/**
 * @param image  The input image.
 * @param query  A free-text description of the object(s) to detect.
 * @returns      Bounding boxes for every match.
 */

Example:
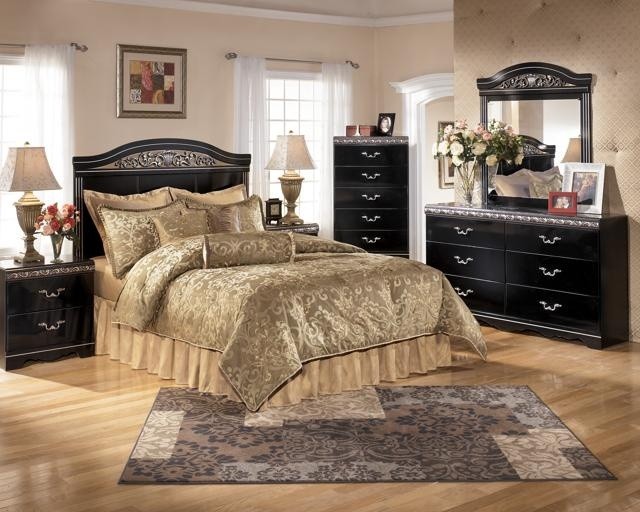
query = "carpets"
[116,384,618,488]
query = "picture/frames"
[376,112,395,136]
[437,121,455,190]
[114,42,189,119]
[546,162,607,219]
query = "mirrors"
[476,59,595,203]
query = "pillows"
[82,182,267,278]
[490,166,563,200]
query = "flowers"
[488,119,524,165]
[31,200,82,248]
[433,120,497,171]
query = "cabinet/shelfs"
[333,134,412,259]
[423,203,630,350]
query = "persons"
[576,177,591,204]
[561,197,572,209]
[554,199,563,209]
[382,117,390,133]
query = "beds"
[65,136,446,410]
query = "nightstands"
[0,259,95,369]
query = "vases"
[49,235,66,264]
[453,163,475,205]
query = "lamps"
[0,141,63,269]
[265,132,317,224]
